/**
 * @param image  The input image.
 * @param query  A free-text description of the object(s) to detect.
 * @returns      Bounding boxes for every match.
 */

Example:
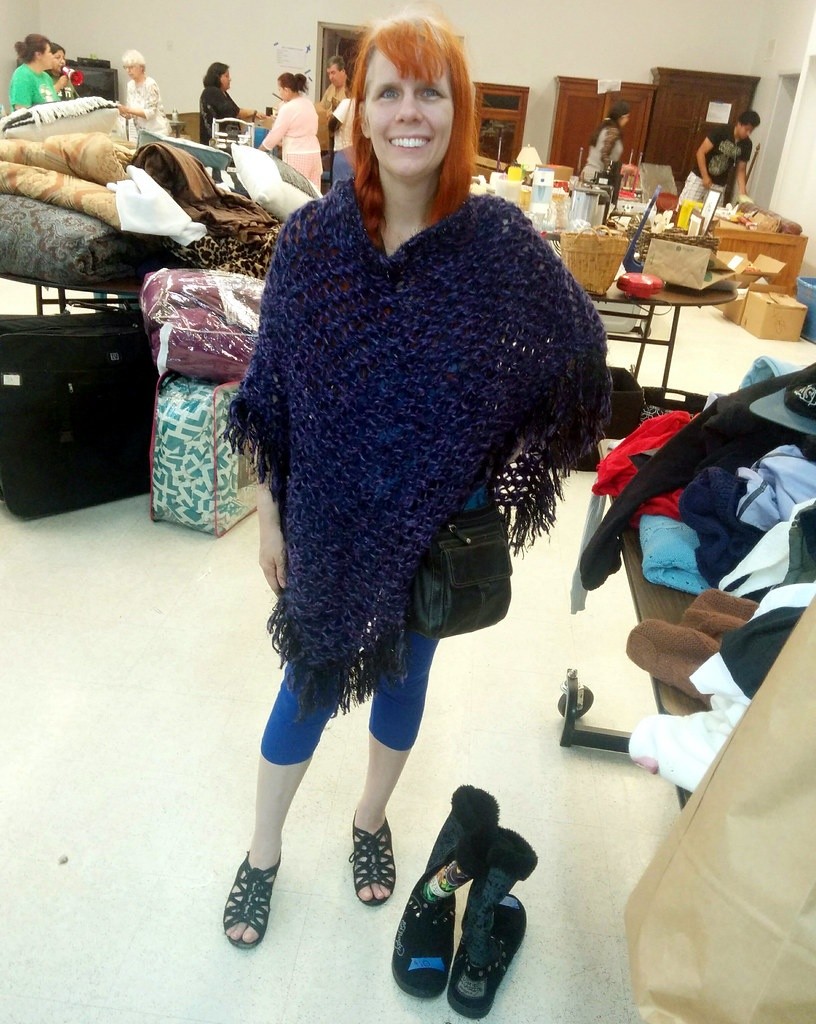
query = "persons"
[315,54,356,188]
[199,62,267,148]
[117,50,172,140]
[220,11,613,950]
[45,42,80,101]
[257,72,323,193]
[676,110,760,214]
[581,100,631,209]
[8,33,60,114]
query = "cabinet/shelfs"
[643,67,762,209]
[473,81,529,165]
[548,77,656,175]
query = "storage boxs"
[551,367,645,473]
[716,249,816,344]
[535,164,574,182]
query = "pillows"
[0,96,121,142]
[231,142,324,221]
[136,130,231,170]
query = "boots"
[448,826,538,1019]
[392,785,499,999]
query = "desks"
[589,273,738,388]
[1,274,142,316]
[558,440,712,811]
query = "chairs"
[643,386,707,414]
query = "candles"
[498,137,501,160]
[636,152,643,173]
[628,149,634,165]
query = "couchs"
[707,202,809,297]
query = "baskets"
[561,225,629,296]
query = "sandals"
[348,811,395,907]
[223,847,282,949]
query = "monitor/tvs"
[73,66,119,103]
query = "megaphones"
[61,66,84,86]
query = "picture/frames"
[700,188,723,236]
[688,210,705,237]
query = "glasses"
[123,65,138,70]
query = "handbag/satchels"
[142,265,267,380]
[408,504,512,637]
[151,370,258,537]
[1,298,158,522]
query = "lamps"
[517,144,542,172]
[577,147,583,173]
